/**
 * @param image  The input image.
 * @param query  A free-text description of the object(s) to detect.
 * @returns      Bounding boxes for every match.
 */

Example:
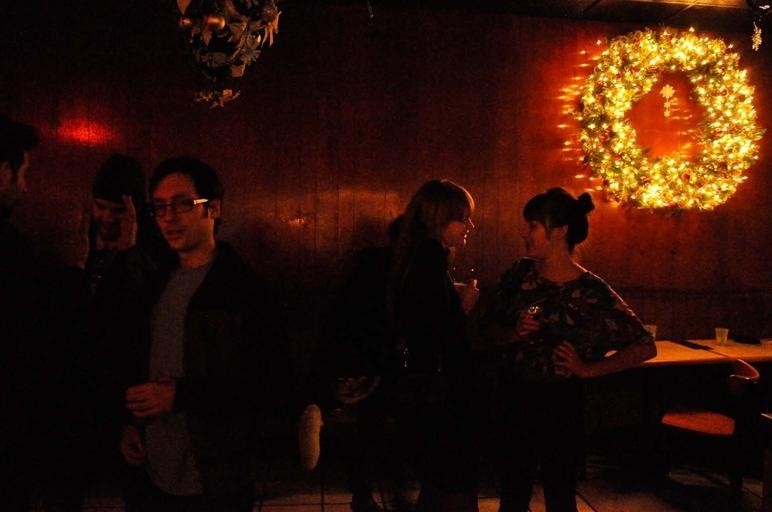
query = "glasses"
[154,196,211,218]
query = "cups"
[715,327,728,346]
[528,303,544,344]
[759,338,772,355]
[645,324,657,340]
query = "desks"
[602,338,772,424]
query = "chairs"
[660,360,761,510]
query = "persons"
[474,187,659,511]
[325,214,414,510]
[0,114,107,510]
[103,150,266,511]
[67,157,159,302]
[378,176,493,510]
